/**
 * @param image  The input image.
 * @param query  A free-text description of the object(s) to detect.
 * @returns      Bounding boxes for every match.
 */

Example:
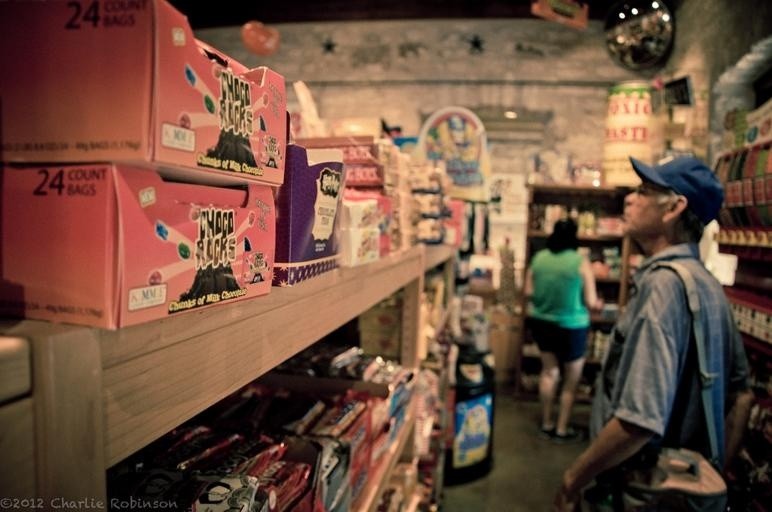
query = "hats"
[629,154,724,225]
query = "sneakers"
[541,422,585,444]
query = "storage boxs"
[0,2,344,332]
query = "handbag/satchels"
[578,446,728,512]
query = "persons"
[549,154,756,512]
[523,215,604,442]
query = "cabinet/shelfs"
[1,238,458,510]
[515,181,629,404]
[713,138,771,512]
